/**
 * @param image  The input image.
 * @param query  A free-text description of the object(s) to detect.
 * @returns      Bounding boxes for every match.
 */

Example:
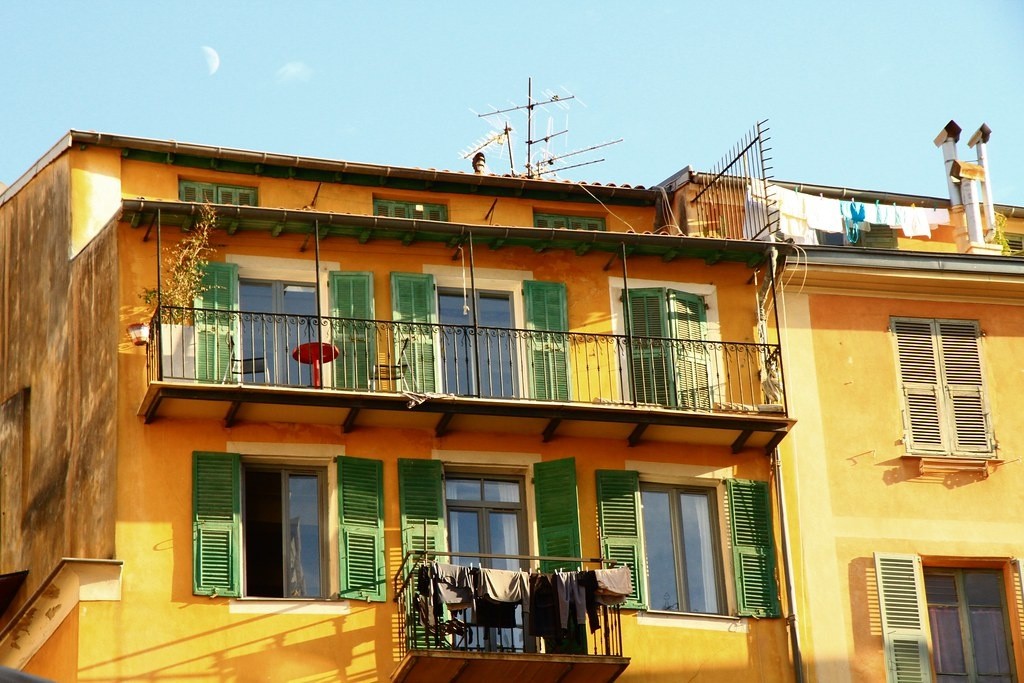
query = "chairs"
[368,337,410,392]
[221,331,270,385]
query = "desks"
[292,342,340,389]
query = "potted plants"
[138,198,228,383]
